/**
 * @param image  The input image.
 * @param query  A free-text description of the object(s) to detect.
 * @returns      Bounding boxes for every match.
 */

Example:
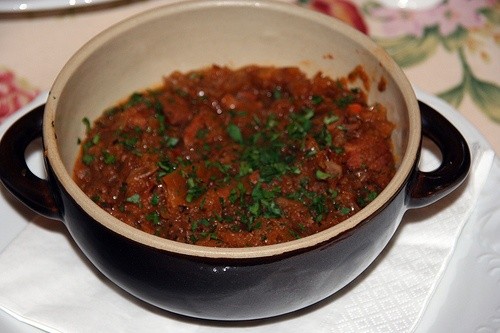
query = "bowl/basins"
[0,0,471,323]
